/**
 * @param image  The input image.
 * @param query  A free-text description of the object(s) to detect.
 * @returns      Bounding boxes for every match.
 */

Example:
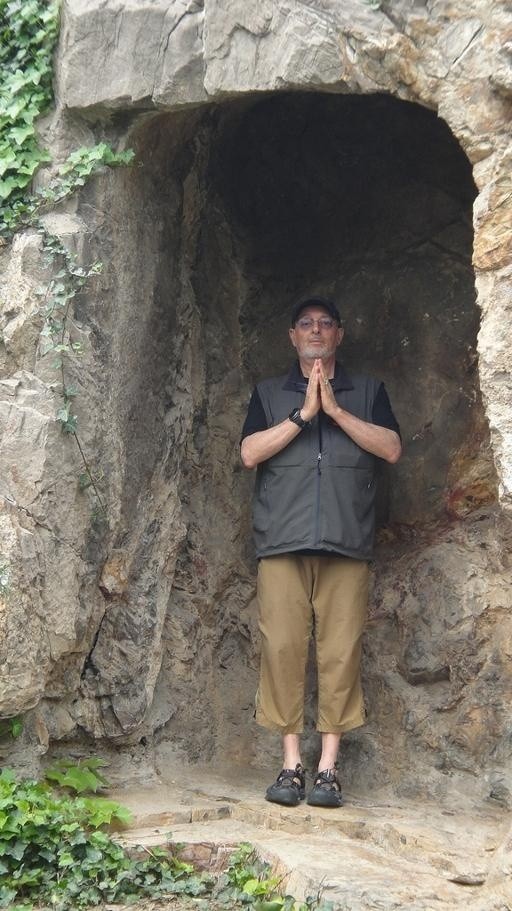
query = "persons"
[236,296,403,809]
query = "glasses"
[301,315,332,330]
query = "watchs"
[288,408,309,430]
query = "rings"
[324,377,329,385]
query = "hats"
[292,297,341,329]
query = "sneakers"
[306,770,341,806]
[265,769,304,805]
